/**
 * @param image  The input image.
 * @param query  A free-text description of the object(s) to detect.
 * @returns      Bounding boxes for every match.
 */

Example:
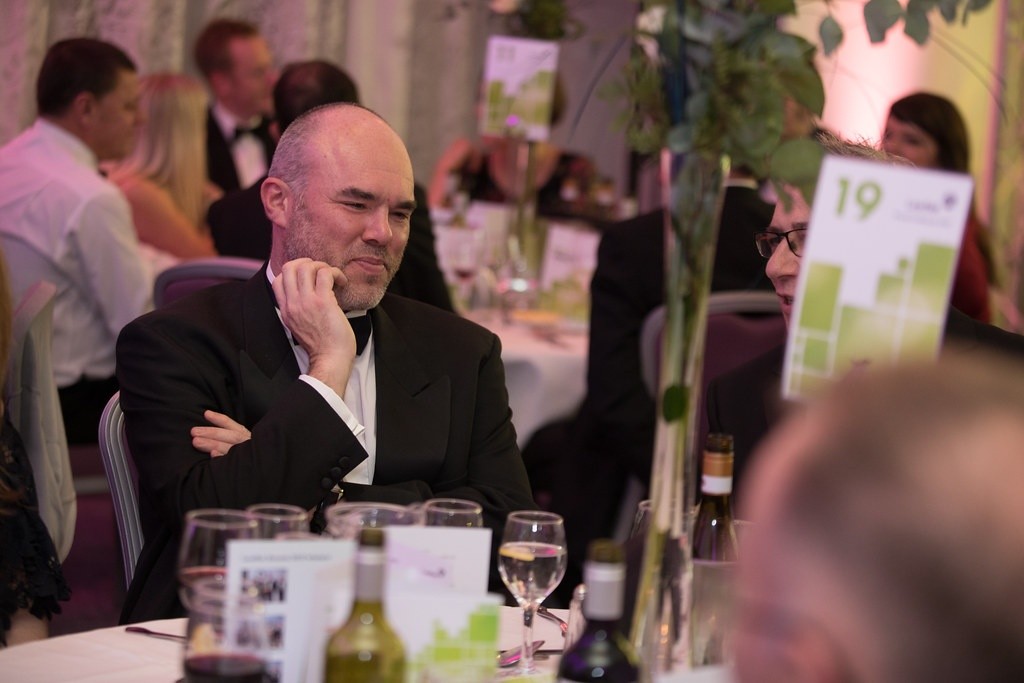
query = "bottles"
[690,432,741,563]
[557,537,645,683]
[323,526,408,683]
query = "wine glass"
[498,511,568,678]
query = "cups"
[183,604,271,683]
[631,500,697,557]
[175,497,484,617]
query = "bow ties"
[291,313,371,356]
[230,122,265,142]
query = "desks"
[0,609,583,682]
[433,200,602,447]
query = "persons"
[102,70,226,263]
[107,102,533,627]
[419,67,1010,607]
[204,59,458,317]
[724,341,1024,683]
[190,19,281,195]
[2,34,179,444]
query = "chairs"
[98,387,145,599]
[638,292,784,454]
[151,259,261,312]
